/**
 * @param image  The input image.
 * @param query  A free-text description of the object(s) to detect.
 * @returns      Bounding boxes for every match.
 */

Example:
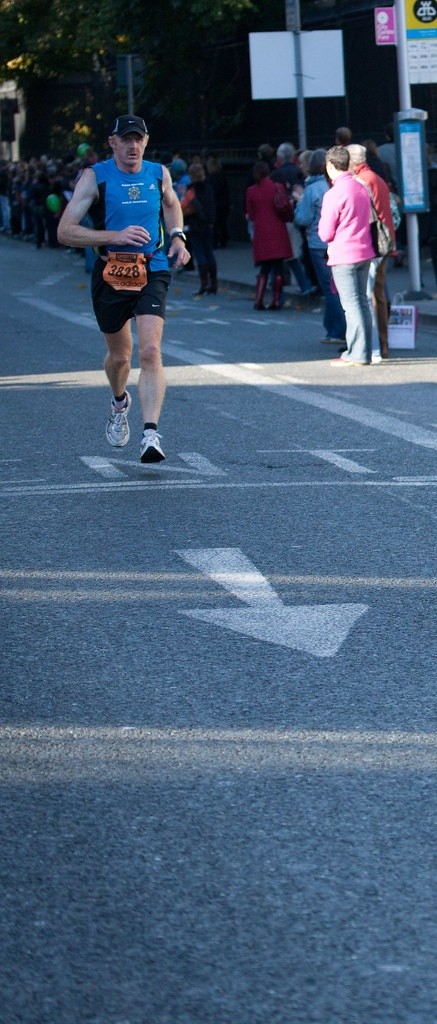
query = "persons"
[57,115,191,463]
[369,123,401,195]
[0,143,202,249]
[166,159,191,201]
[272,142,306,197]
[244,143,274,221]
[278,222,316,309]
[207,156,230,249]
[363,139,396,195]
[335,128,352,145]
[293,149,345,344]
[182,163,218,296]
[346,144,397,359]
[246,161,292,311]
[317,145,382,365]
[293,150,312,182]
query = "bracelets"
[169,227,187,243]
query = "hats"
[110,115,148,138]
[168,159,187,173]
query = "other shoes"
[330,357,367,367]
[369,357,382,364]
[320,337,345,344]
[298,287,313,295]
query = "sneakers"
[139,429,166,464]
[105,390,132,447]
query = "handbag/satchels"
[274,183,293,221]
[369,199,393,258]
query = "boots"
[194,263,209,295]
[207,263,217,294]
[254,274,267,310]
[267,275,283,310]
